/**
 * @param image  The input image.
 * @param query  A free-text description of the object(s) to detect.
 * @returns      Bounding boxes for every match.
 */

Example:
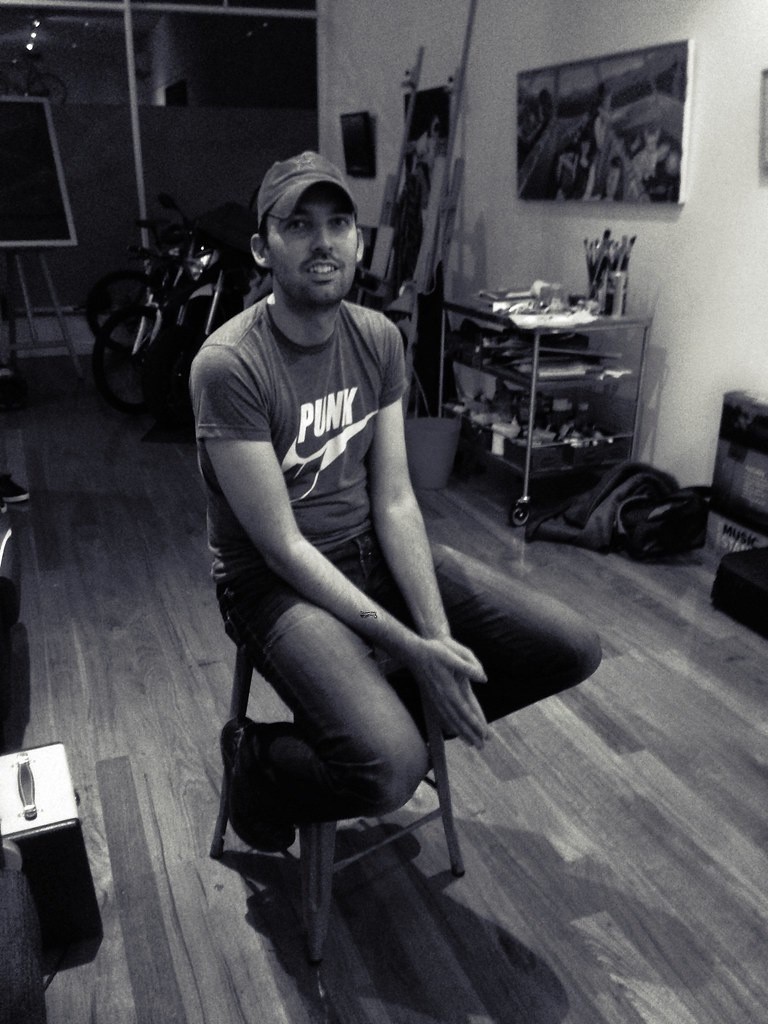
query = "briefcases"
[0,742,101,936]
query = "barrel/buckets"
[402,416,461,491]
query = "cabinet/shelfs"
[436,296,653,527]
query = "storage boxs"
[707,546,768,642]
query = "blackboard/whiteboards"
[0,95,80,248]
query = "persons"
[189,152,603,853]
[0,293,30,513]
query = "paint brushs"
[583,228,639,271]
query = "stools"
[204,639,466,967]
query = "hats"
[257,152,358,231]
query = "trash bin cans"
[404,417,463,489]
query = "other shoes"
[0,474,30,513]
[220,719,295,853]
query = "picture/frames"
[497,34,700,209]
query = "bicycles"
[76,189,220,418]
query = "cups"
[599,271,625,317]
